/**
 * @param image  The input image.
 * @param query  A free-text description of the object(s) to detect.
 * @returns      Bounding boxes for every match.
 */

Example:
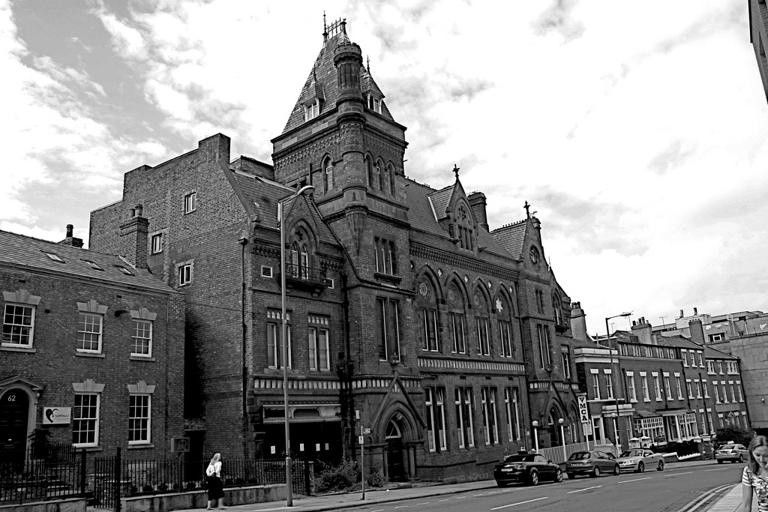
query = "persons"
[206,453,227,510]
[742,436,768,512]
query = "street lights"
[605,309,634,452]
[274,185,316,509]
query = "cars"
[568,450,621,478]
[490,452,564,488]
[714,442,749,463]
[615,448,666,476]
